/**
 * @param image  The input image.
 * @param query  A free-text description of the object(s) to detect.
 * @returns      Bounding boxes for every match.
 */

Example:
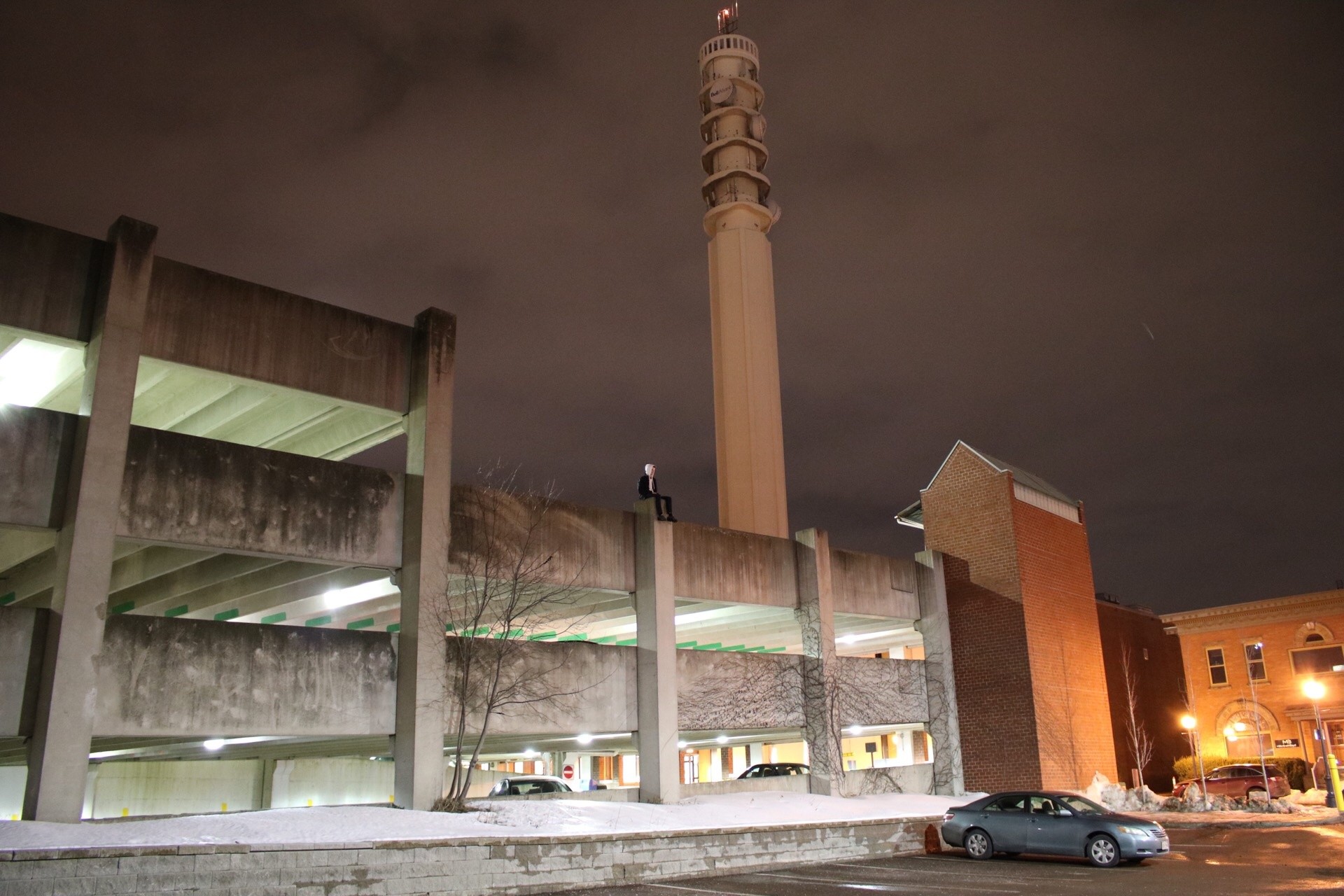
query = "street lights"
[1301,678,1336,808]
[1181,713,1202,778]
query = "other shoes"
[658,515,667,521]
[667,516,678,522]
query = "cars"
[488,776,573,798]
[734,763,813,781]
[942,789,1169,866]
[1171,764,1291,802]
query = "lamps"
[1303,619,1316,631]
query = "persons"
[636,462,681,524]
